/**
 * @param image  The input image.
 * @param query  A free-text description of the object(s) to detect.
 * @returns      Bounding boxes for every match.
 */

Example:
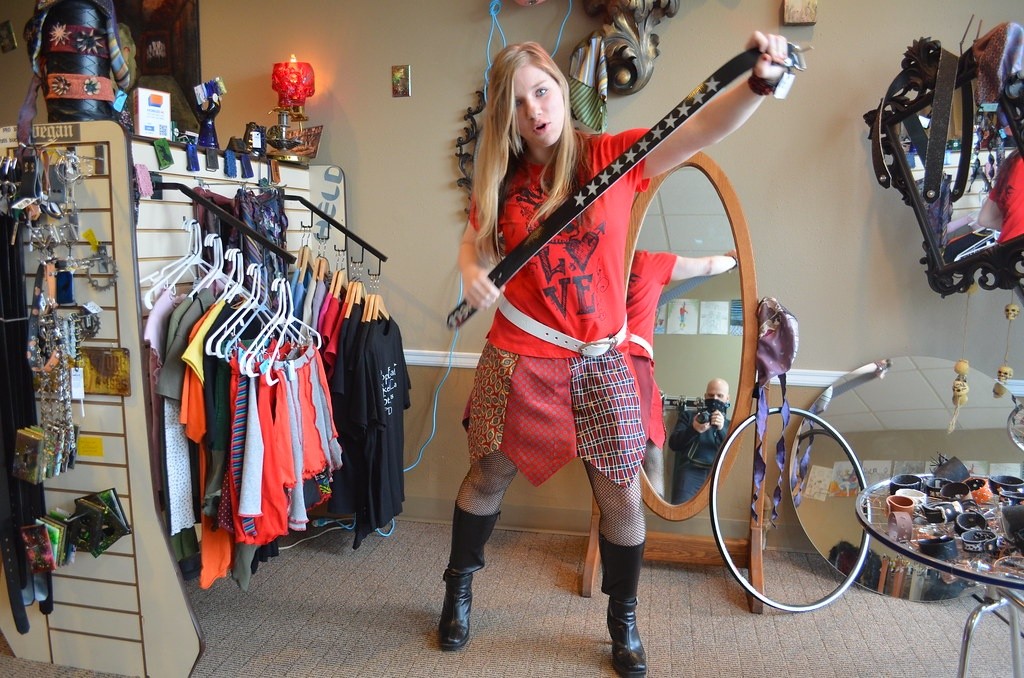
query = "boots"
[607,596,646,678]
[438,568,473,651]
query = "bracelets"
[724,255,737,272]
[747,68,776,96]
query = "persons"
[435,30,789,677]
[667,377,732,505]
[626,248,741,499]
[978,143,1024,242]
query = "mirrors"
[790,354,1024,602]
[863,22,1023,305]
[579,153,767,613]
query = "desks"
[856,473,1024,678]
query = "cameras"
[698,411,713,423]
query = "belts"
[498,294,628,358]
[0,147,59,635]
[630,334,653,360]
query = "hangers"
[140,217,322,387]
[295,225,391,323]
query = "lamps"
[267,55,314,163]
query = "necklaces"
[38,311,75,465]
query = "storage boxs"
[132,87,172,141]
